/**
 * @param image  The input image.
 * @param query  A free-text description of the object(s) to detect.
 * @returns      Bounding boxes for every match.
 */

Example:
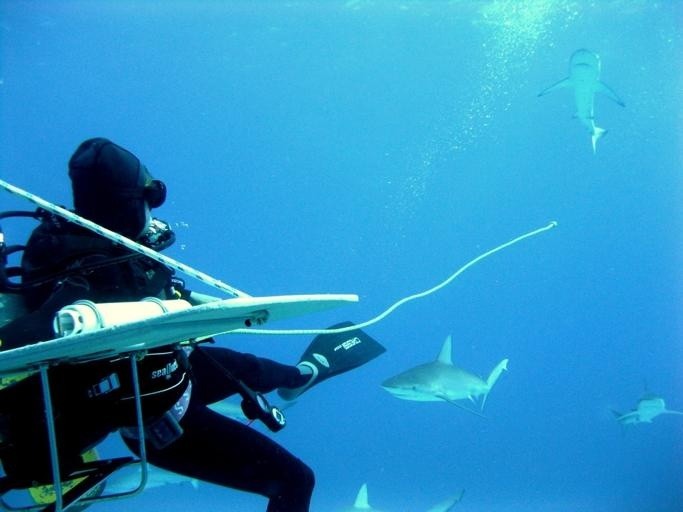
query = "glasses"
[144,180,166,208]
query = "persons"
[19,136,388,512]
